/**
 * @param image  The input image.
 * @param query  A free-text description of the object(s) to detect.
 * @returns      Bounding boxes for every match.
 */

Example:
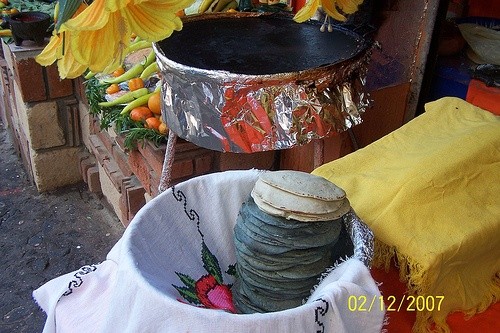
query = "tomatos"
[105,67,170,135]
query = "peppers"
[97,49,161,115]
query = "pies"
[231,171,351,317]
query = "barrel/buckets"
[125,169,375,331]
[4,11,51,47]
